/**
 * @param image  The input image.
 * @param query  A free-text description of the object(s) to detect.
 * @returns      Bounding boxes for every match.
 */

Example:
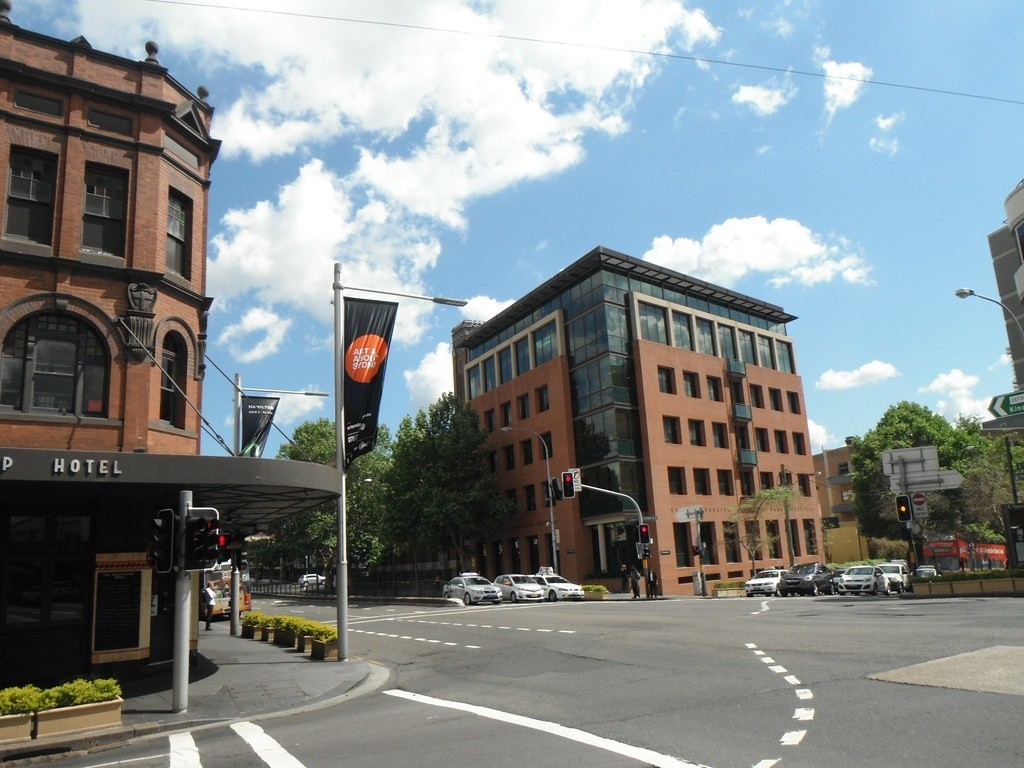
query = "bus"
[200,558,251,617]
[921,533,1008,571]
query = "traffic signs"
[987,390,1024,418]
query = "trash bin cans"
[692,571,708,595]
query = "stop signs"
[911,493,926,506]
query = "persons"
[629,565,641,599]
[620,565,628,592]
[204,580,217,630]
[647,568,658,599]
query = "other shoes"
[633,595,640,599]
[651,596,657,600]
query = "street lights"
[230,373,329,635]
[331,262,467,662]
[501,427,558,574]
[784,472,821,567]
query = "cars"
[833,563,942,595]
[442,576,502,605]
[494,574,545,603]
[528,574,585,602]
[745,569,784,597]
[298,574,327,587]
[778,562,835,596]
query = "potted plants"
[0,685,43,740]
[32,677,124,738]
[272,615,293,644]
[951,571,983,594]
[260,615,289,642]
[298,621,322,653]
[929,575,953,594]
[711,581,746,597]
[980,570,1015,592]
[582,585,596,598]
[285,617,309,647]
[590,585,606,599]
[241,612,269,639]
[311,626,338,659]
[1010,568,1024,593]
[909,576,931,595]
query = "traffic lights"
[188,507,229,571]
[895,495,912,522]
[151,508,175,573]
[560,472,575,498]
[639,524,650,544]
